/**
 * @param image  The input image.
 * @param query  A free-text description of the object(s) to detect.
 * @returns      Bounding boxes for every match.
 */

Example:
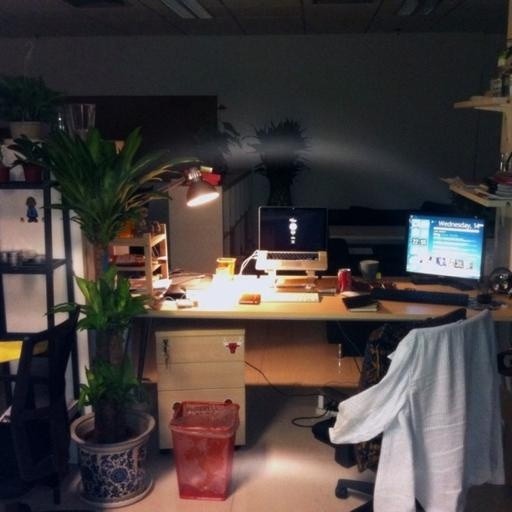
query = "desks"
[126,265,512,454]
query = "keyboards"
[259,292,320,303]
[371,288,470,305]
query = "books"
[128,275,172,303]
[342,295,379,312]
[473,176,512,200]
[115,254,158,265]
[240,294,261,304]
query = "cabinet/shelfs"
[447,91,512,210]
[2,137,84,507]
[99,224,169,300]
[166,167,255,274]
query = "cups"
[217,257,236,278]
[360,259,379,282]
[499,151,512,175]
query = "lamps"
[141,165,221,209]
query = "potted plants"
[0,73,64,141]
[240,116,313,205]
[0,100,210,510]
[7,133,48,184]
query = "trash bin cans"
[168,399,240,501]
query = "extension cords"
[315,399,338,417]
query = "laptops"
[256,206,328,271]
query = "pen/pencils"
[499,152,512,174]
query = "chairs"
[334,305,495,512]
[0,303,83,506]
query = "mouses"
[477,294,492,304]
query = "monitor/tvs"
[404,213,487,283]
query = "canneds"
[338,268,352,294]
